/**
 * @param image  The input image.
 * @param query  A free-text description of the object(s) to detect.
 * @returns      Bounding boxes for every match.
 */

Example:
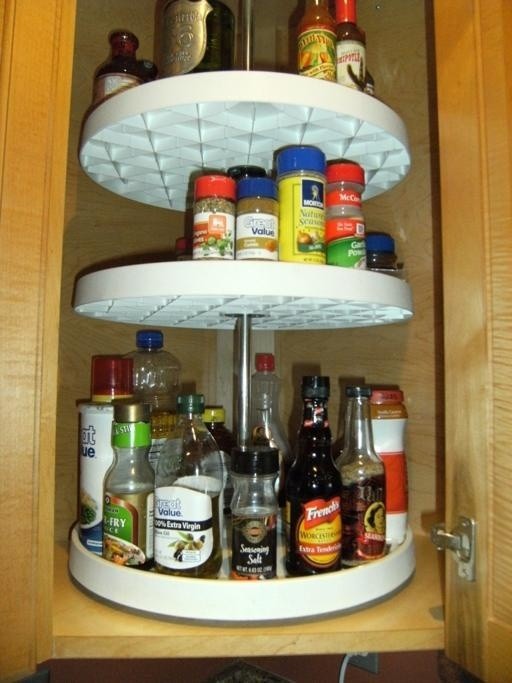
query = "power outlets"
[346,651,379,674]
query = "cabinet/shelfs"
[0,0,510,683]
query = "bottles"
[192,174,237,260]
[90,354,134,404]
[121,328,185,473]
[174,237,192,261]
[297,0,337,84]
[368,387,410,553]
[275,145,328,265]
[157,1,235,80]
[229,443,286,581]
[237,175,280,262]
[333,0,367,91]
[364,233,398,278]
[200,405,241,539]
[335,384,388,569]
[153,392,223,578]
[76,400,116,557]
[92,30,145,111]
[247,352,294,470]
[283,374,345,576]
[324,162,368,273]
[226,165,268,180]
[101,398,158,570]
